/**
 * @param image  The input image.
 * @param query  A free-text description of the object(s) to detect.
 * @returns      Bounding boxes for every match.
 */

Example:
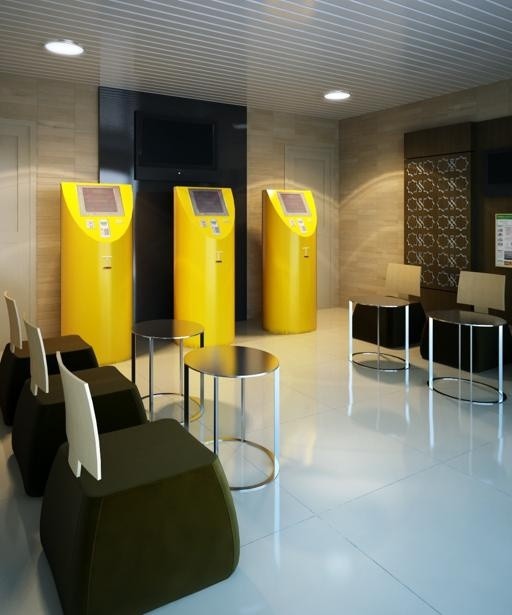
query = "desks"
[425,309,508,406]
[130,319,205,427]
[348,295,410,372]
[184,345,280,494]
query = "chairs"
[40,350,241,615]
[11,319,149,499]
[352,263,427,350]
[419,270,511,374]
[0,290,99,427]
